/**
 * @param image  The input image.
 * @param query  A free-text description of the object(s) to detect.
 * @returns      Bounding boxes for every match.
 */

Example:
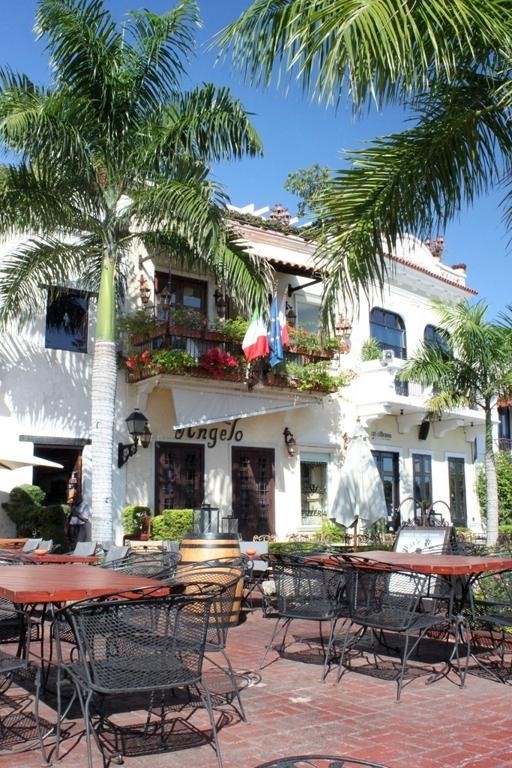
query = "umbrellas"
[326,414,388,552]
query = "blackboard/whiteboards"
[385,527,452,601]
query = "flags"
[268,292,285,367]
[278,291,290,346]
[241,294,271,363]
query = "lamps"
[118,411,147,469]
[284,427,298,457]
[140,423,152,449]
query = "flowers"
[127,308,358,392]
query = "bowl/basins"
[35,549,48,555]
